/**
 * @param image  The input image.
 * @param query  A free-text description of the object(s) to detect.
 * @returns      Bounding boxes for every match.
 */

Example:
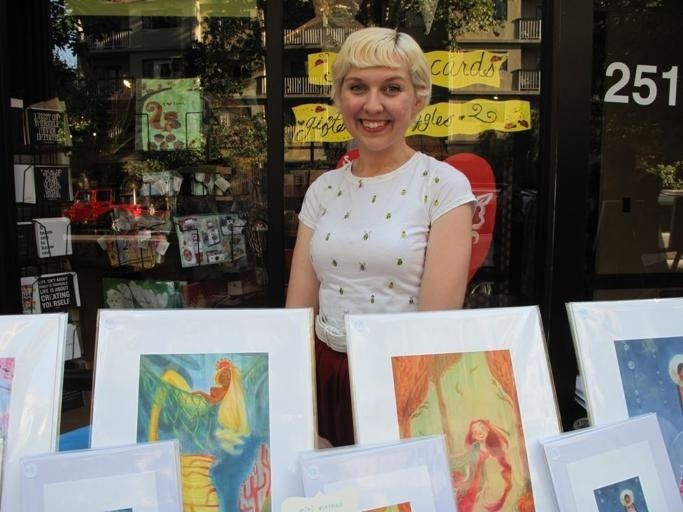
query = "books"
[13,104,85,363]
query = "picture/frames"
[19,439,181,512]
[565,297,682,505]
[343,303,565,512]
[541,409,683,511]
[88,308,318,512]
[0,313,68,511]
[299,433,460,512]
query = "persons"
[283,24,478,453]
